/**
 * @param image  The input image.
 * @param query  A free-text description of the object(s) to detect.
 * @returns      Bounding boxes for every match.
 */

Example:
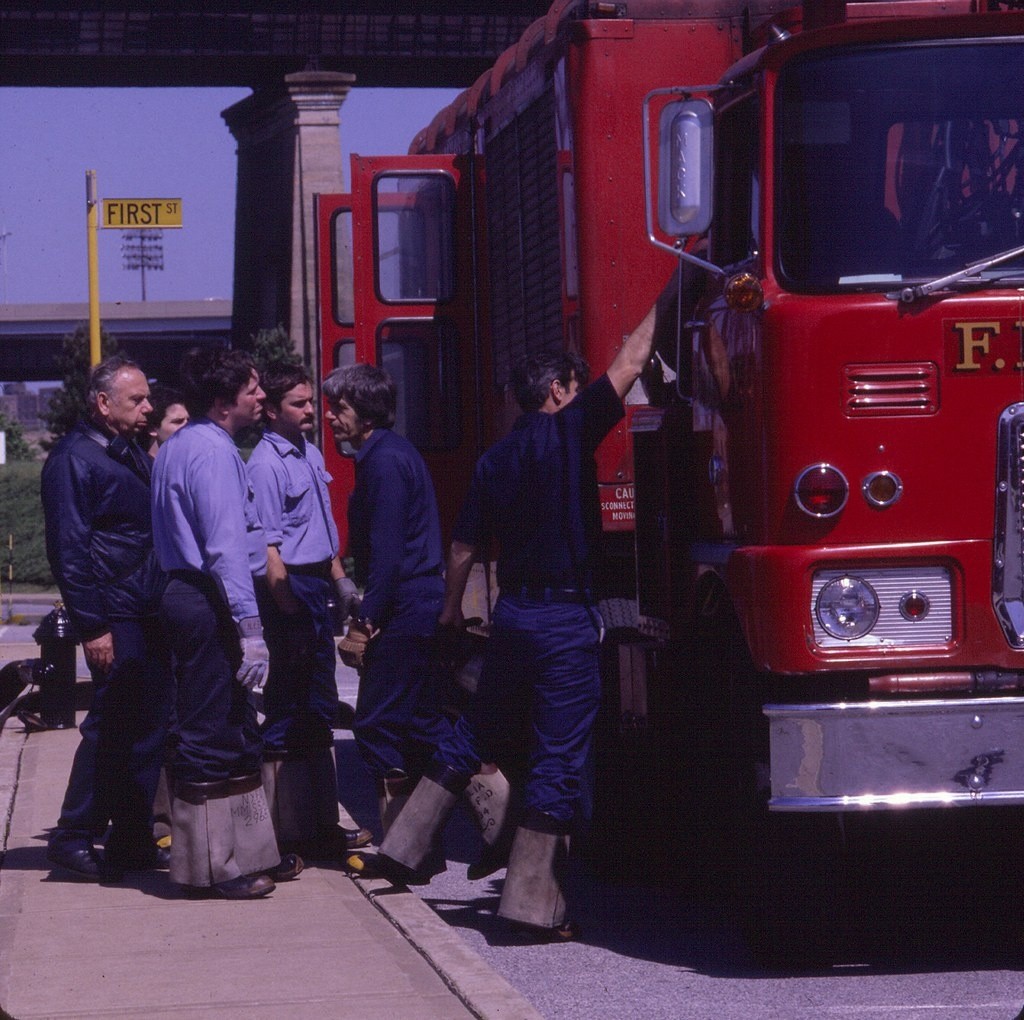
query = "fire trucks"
[313,0,1024,886]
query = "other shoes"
[346,853,416,881]
[511,920,572,942]
[376,856,429,885]
[467,840,513,880]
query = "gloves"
[338,620,369,670]
[236,637,269,690]
[333,578,362,621]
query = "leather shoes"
[154,834,172,849]
[104,840,171,868]
[47,839,124,882]
[296,841,321,861]
[246,855,304,881]
[180,874,276,899]
[323,823,373,847]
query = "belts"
[500,585,585,603]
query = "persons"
[375,248,707,936]
[43,342,370,897]
[318,359,516,876]
